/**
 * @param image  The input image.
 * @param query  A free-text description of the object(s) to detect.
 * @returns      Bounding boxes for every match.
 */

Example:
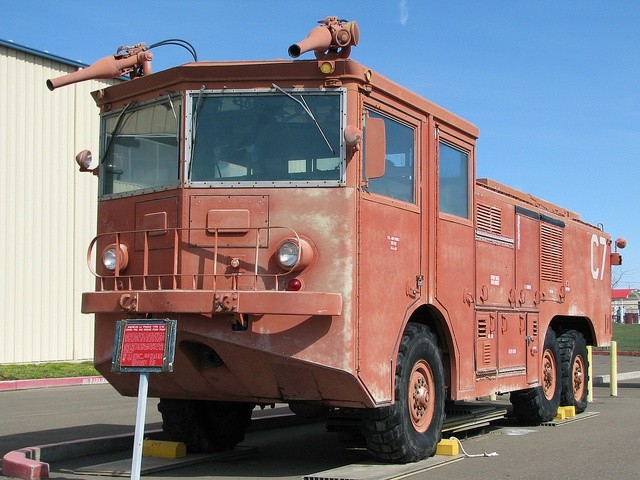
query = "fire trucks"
[44,15,629,463]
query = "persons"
[616,307,621,323]
